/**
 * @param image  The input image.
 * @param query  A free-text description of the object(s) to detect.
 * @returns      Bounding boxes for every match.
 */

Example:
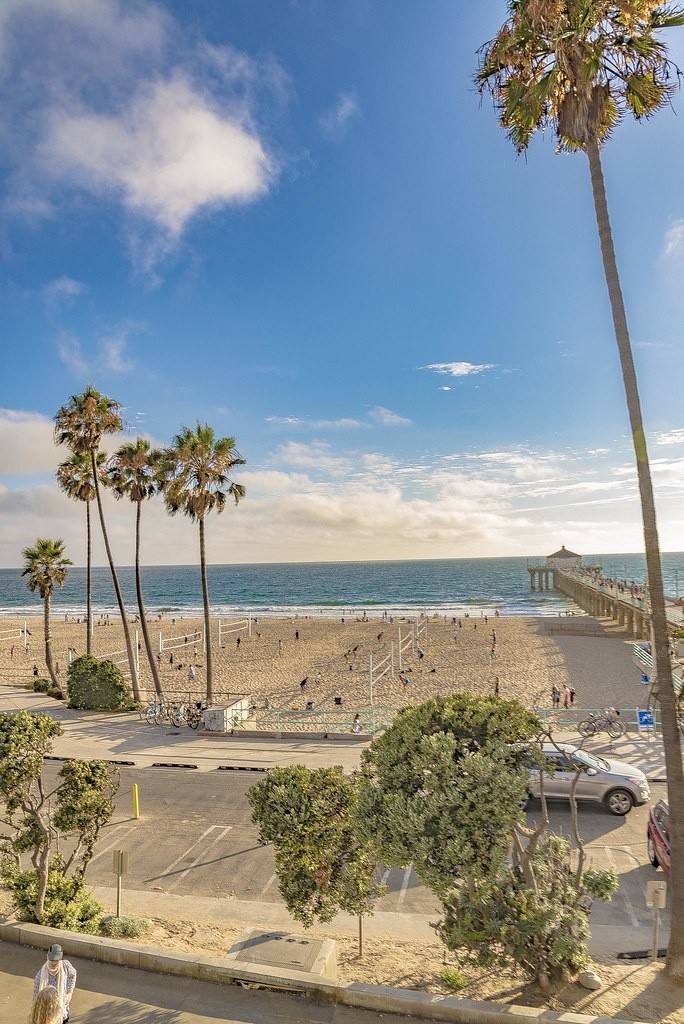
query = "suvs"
[646,798,671,881]
[497,742,651,816]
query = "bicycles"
[138,699,204,730]
[577,709,627,739]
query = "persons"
[28,943,77,1024]
[10,570,684,733]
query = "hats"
[48,944,63,960]
